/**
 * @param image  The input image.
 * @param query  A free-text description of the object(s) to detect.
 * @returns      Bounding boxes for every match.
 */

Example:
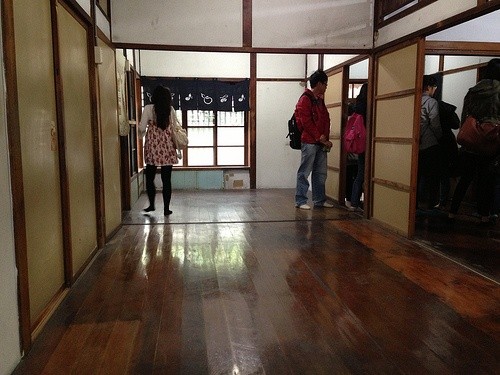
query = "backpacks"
[344,110,367,153]
[287,92,316,150]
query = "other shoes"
[448,212,457,218]
[144,205,155,212]
[164,209,172,215]
[432,201,441,208]
[299,204,311,209]
[349,204,363,211]
[323,201,334,207]
[345,200,351,207]
[482,215,497,228]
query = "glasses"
[323,82,328,87]
[432,84,437,89]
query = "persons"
[294,70,334,209]
[344,82,368,209]
[139,86,178,215]
[418,59,500,229]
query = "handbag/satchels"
[457,114,500,150]
[170,106,188,148]
